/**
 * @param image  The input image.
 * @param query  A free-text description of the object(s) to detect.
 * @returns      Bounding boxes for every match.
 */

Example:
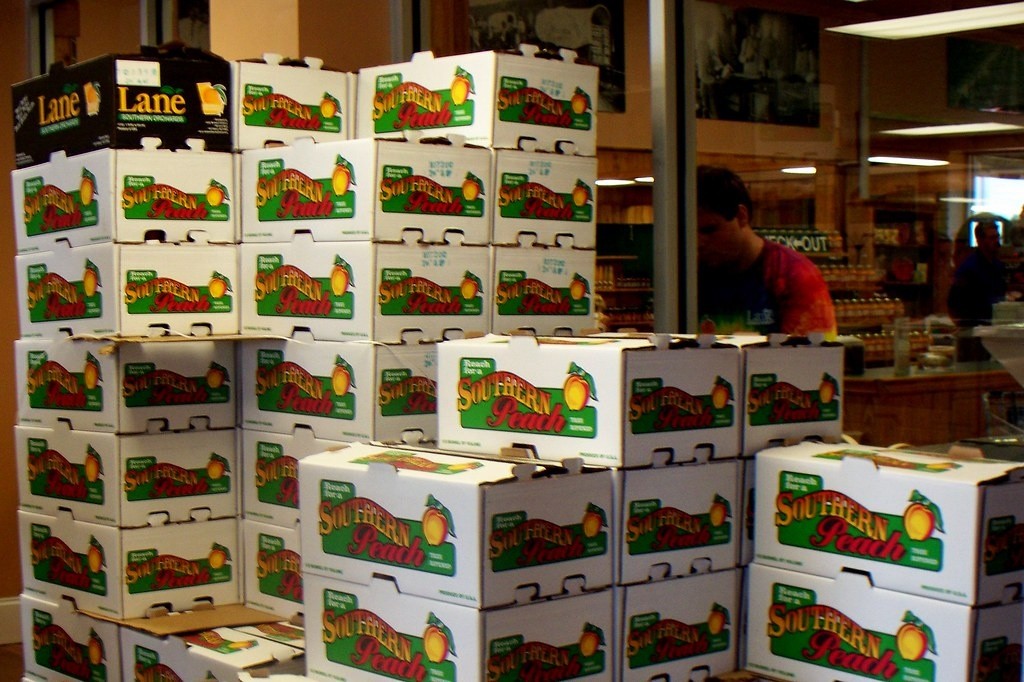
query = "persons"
[945,222,1008,362]
[696,165,838,335]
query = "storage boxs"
[241,137,493,245]
[241,519,302,618]
[18,510,240,620]
[20,573,739,682]
[298,441,614,611]
[15,242,239,339]
[241,428,349,530]
[241,241,491,344]
[437,330,741,469]
[355,44,599,156]
[240,340,437,444]
[13,425,238,528]
[12,147,237,254]
[737,437,1024,682]
[14,339,236,434]
[492,149,598,334]
[10,39,231,168]
[613,466,741,585]
[591,333,844,458]
[232,53,348,152]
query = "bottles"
[604,306,644,322]
[816,263,885,292]
[835,294,929,375]
[596,266,614,289]
[754,229,842,260]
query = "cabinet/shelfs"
[597,253,654,326]
[876,202,939,313]
